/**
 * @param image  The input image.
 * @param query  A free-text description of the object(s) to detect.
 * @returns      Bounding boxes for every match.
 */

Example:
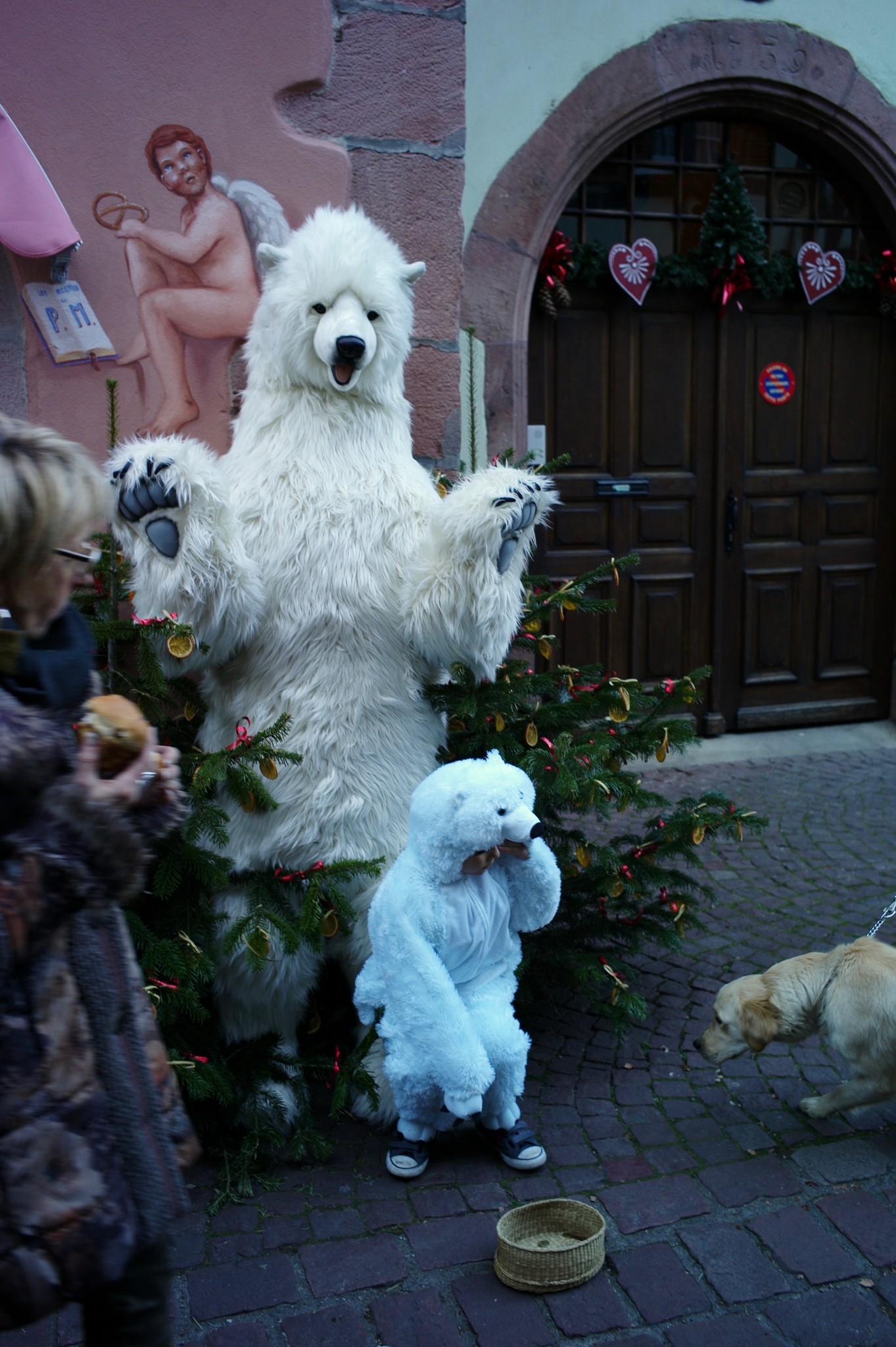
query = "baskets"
[493,1198,607,1294]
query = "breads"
[74,688,151,755]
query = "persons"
[351,749,565,1178]
[0,412,207,1347]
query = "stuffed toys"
[105,199,577,1124]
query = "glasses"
[52,540,103,565]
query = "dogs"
[692,934,896,1120]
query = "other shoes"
[385,1131,432,1178]
[492,1120,547,1171]
